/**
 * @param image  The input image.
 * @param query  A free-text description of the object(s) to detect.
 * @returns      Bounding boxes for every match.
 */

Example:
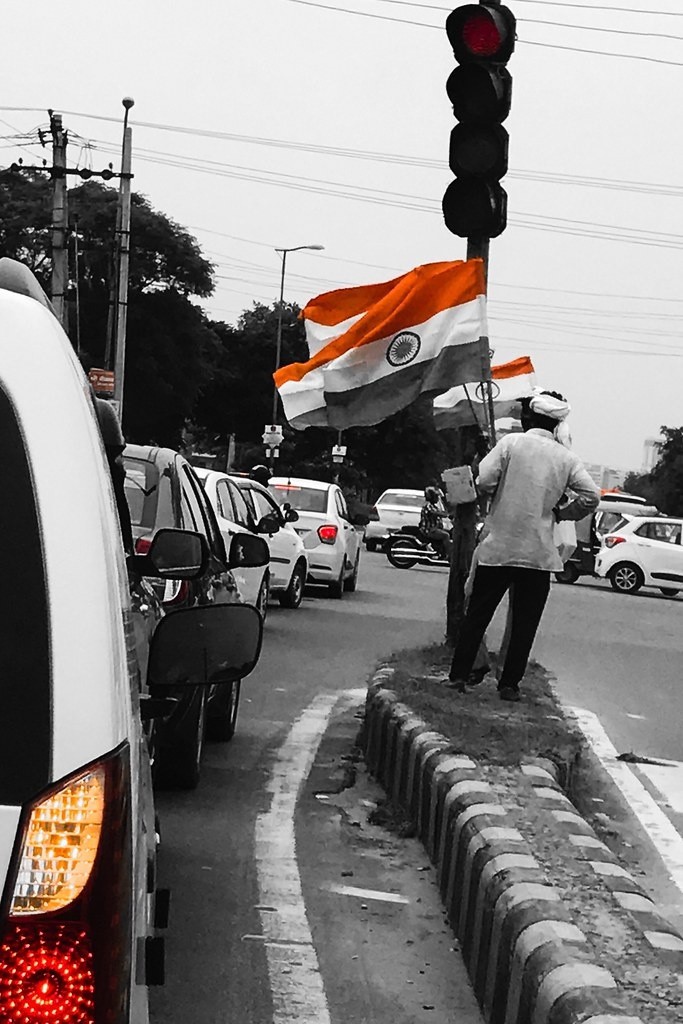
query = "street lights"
[269,244,328,478]
[111,96,136,430]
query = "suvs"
[0,259,267,1024]
[551,493,666,584]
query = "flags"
[273,258,491,430]
[432,357,540,431]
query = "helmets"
[425,486,439,500]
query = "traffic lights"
[442,1,511,239]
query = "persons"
[416,486,454,555]
[249,456,272,490]
[76,350,135,555]
[443,391,600,701]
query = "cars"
[229,474,308,608]
[593,513,682,595]
[114,444,272,789]
[120,467,282,623]
[262,476,373,599]
[365,489,455,552]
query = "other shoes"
[465,664,492,685]
[435,678,466,694]
[501,687,520,702]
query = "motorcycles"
[382,524,451,570]
